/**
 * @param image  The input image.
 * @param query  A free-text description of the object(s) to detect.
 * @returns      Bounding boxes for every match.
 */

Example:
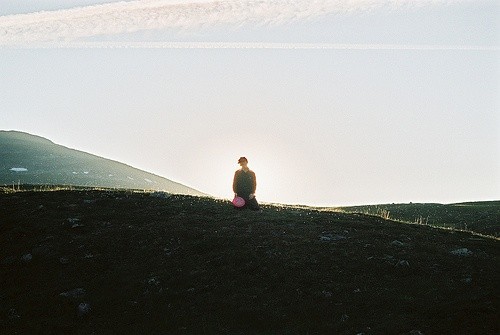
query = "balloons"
[233,197,245,207]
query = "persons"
[233,156,259,210]
